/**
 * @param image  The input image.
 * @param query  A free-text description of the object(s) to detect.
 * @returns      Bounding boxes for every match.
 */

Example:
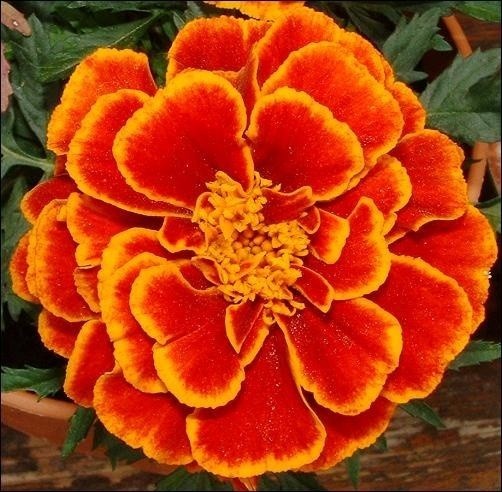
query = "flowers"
[0,0,500,491]
